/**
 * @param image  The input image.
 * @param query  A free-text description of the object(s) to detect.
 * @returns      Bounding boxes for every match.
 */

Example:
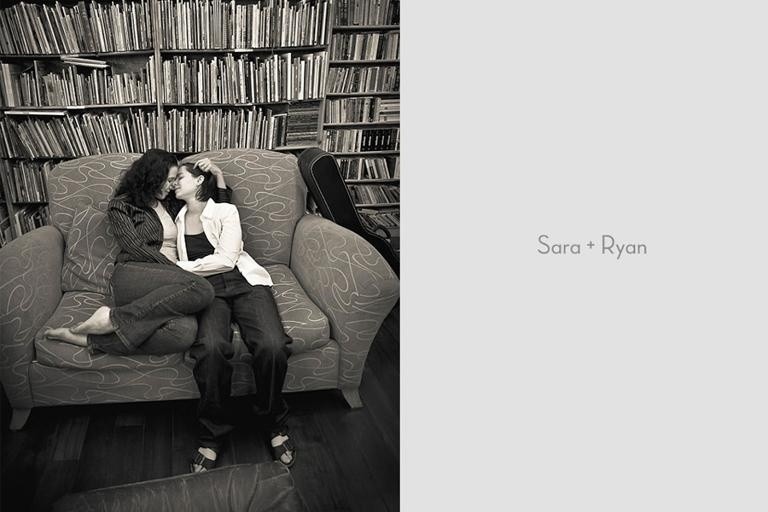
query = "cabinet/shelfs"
[0,1,400,241]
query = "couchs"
[0,148,400,432]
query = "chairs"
[298,147,400,281]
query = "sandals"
[271,436,297,467]
[190,447,218,473]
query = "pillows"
[61,202,120,295]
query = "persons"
[0,0,400,247]
[43,148,232,358]
[172,162,297,474]
